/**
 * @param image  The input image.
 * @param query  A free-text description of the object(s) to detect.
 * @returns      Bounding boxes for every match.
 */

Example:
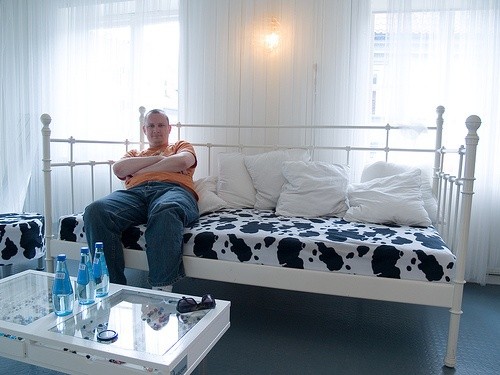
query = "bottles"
[56,297,110,342]
[52,254,74,316]
[77,247,97,305]
[91,241,110,297]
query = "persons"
[84,109,200,293]
[146,305,169,330]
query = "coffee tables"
[0,269,230,375]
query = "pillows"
[192,149,448,228]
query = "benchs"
[40,106,480,368]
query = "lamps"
[262,16,283,57]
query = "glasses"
[176,294,216,313]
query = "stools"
[0,212,45,278]
